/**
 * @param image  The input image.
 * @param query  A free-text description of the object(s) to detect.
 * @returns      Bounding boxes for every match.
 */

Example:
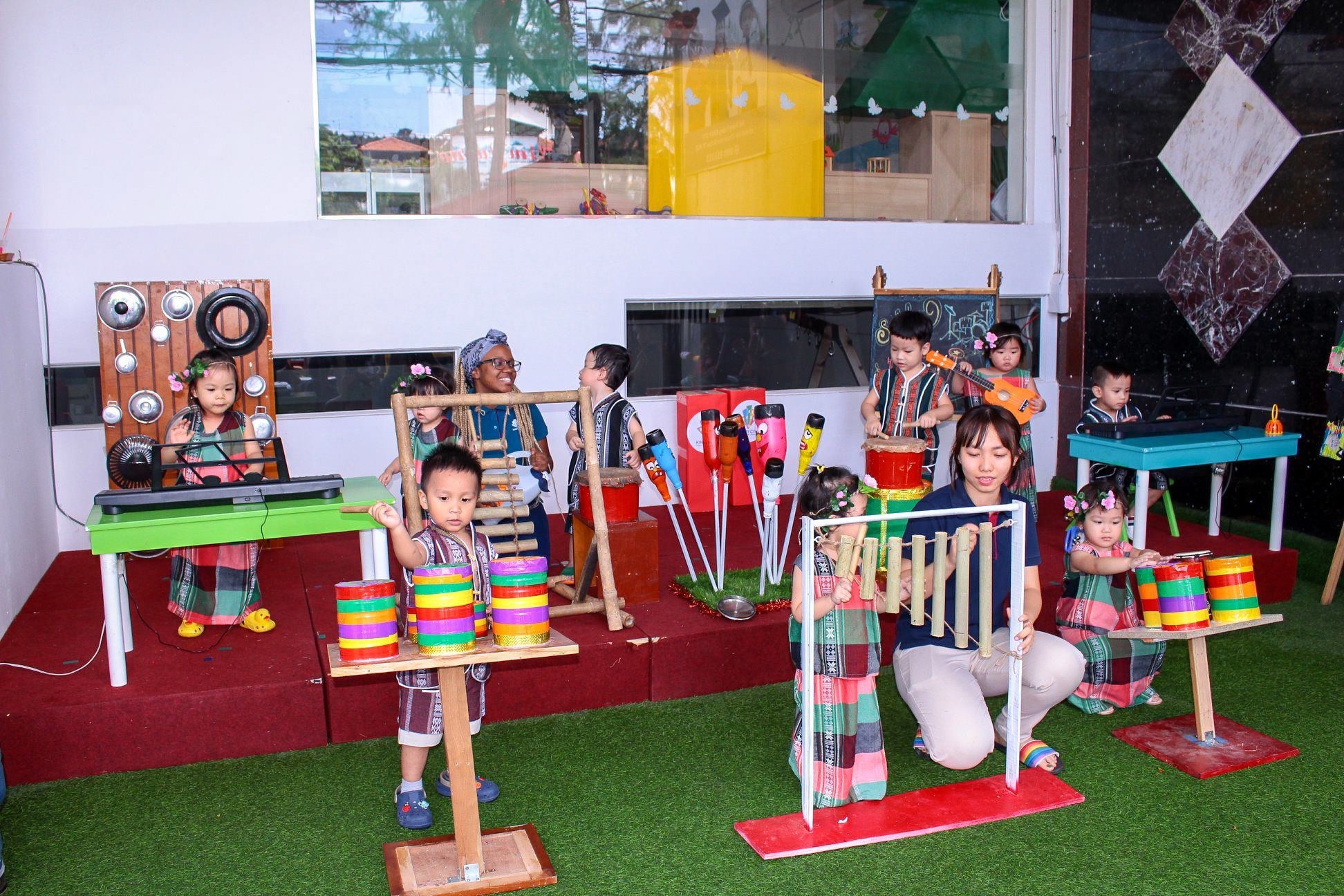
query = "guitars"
[925,350,1036,426]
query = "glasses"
[476,358,522,372]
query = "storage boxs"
[716,386,767,507]
[677,390,730,512]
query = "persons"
[369,442,501,829]
[860,311,955,494]
[787,466,927,809]
[440,330,554,580]
[1074,362,1170,543]
[892,405,1088,775]
[1056,481,1177,716]
[161,350,275,638]
[952,322,1046,523]
[378,367,479,538]
[560,343,645,583]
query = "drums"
[861,437,929,489]
[574,467,644,523]
[1134,548,1261,631]
[334,556,550,664]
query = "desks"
[1069,427,1301,553]
[326,628,580,894]
[1107,615,1298,779]
[85,477,397,687]
[320,171,430,215]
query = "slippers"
[913,729,932,760]
[995,739,1064,775]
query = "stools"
[570,507,659,609]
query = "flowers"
[837,473,878,507]
[1064,491,1115,512]
[392,363,430,392]
[973,332,997,350]
[167,360,209,391]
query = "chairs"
[1066,477,1179,547]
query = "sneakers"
[1123,514,1134,540]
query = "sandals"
[239,608,276,633]
[1097,706,1114,715]
[561,566,574,584]
[394,783,432,829]
[1144,693,1162,705]
[436,771,500,803]
[177,618,204,637]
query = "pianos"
[1081,383,1240,440]
[94,436,344,516]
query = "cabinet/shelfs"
[429,45,990,221]
